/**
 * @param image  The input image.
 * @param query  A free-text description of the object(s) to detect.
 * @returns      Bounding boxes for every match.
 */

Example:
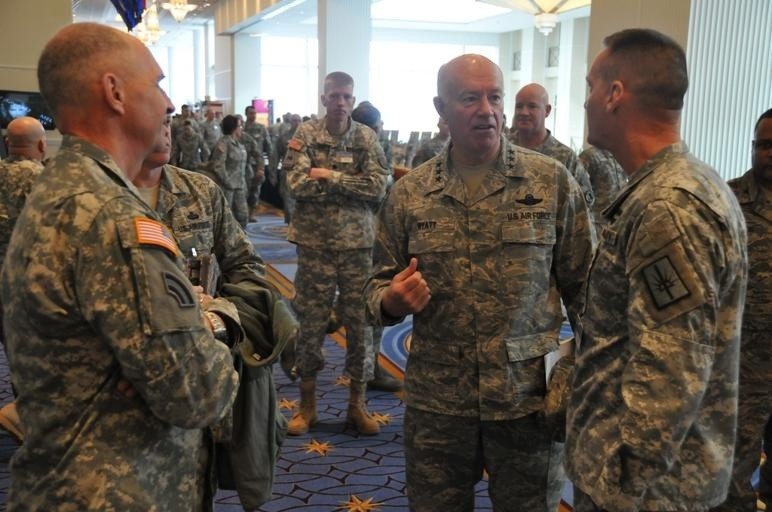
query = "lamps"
[124,0,199,47]
[474,0,594,36]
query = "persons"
[565,26,747,512]
[728,108,772,512]
[506,83,594,227]
[360,53,590,512]
[412,119,452,171]
[578,147,627,238]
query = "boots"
[370,359,402,391]
[347,379,381,435]
[286,380,319,435]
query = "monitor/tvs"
[0,89,56,130]
